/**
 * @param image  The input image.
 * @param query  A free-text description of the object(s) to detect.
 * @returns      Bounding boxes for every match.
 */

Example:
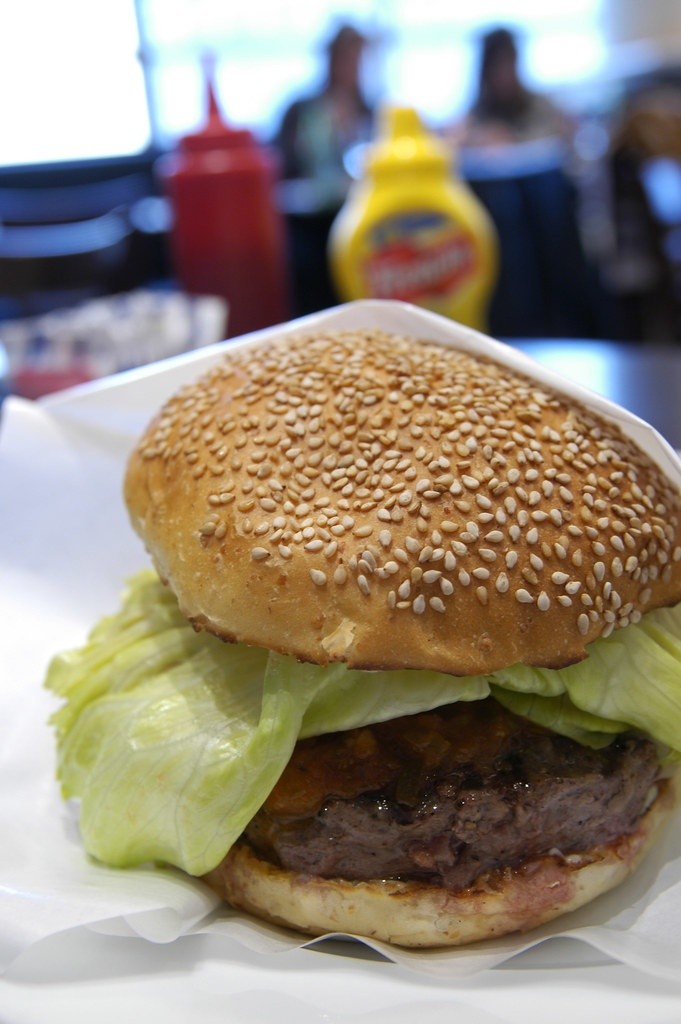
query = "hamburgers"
[44,333,681,949]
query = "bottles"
[329,107,496,333]
[171,85,286,339]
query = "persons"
[591,63,681,338]
[281,24,373,179]
[451,29,575,149]
